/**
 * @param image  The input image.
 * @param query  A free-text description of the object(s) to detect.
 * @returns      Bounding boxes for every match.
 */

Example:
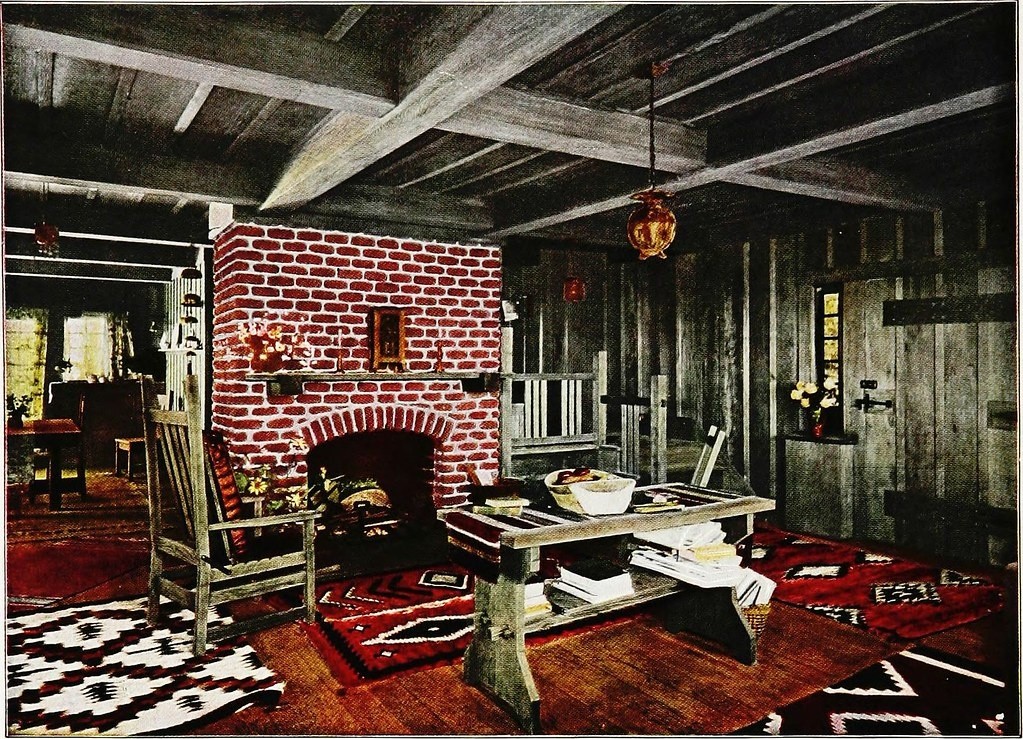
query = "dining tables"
[437,481,779,735]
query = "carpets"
[302,515,1018,689]
[725,638,1021,734]
[8,589,289,737]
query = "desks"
[5,419,86,514]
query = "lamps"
[562,243,589,304]
[623,61,683,265]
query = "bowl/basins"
[567,476,637,516]
[543,465,612,510]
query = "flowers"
[790,381,842,422]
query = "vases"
[7,415,24,429]
[811,424,823,438]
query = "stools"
[114,435,146,478]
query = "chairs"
[143,400,325,653]
